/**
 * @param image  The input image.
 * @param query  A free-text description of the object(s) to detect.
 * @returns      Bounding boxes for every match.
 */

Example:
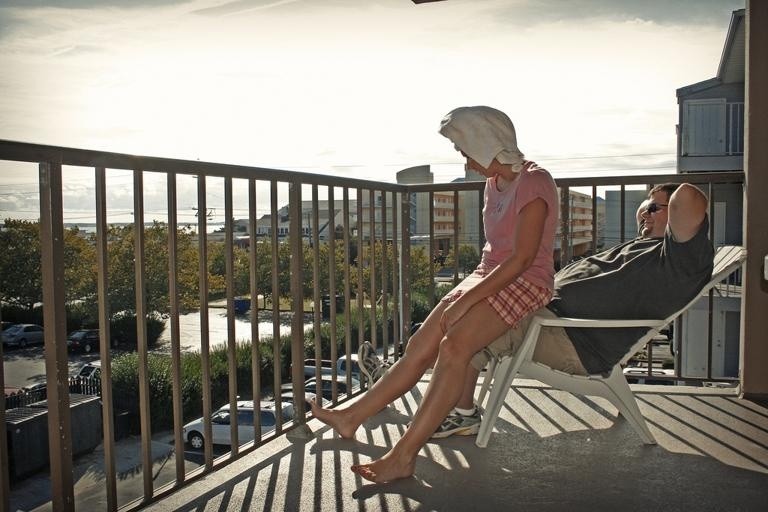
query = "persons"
[311,106,559,484]
[358,182,714,440]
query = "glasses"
[645,201,668,214]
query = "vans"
[179,398,297,448]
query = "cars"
[1,324,46,349]
[66,328,117,354]
[270,352,401,414]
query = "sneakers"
[357,340,391,389]
[405,403,482,439]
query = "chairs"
[472,244,749,448]
